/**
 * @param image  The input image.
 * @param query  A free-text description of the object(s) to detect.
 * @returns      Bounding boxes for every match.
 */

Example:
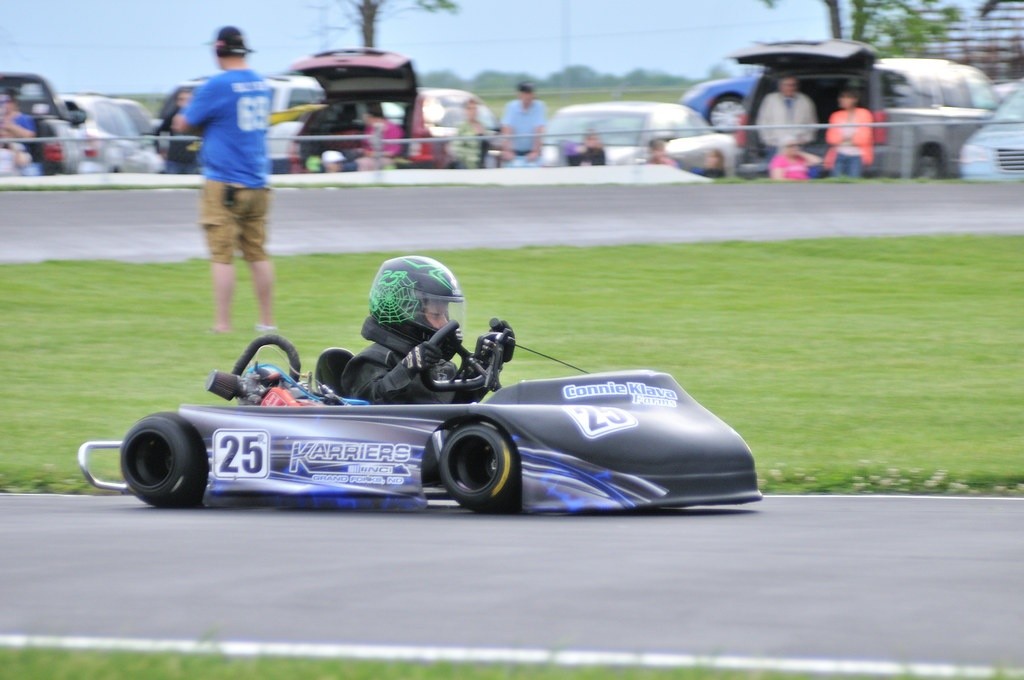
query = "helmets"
[361,256,465,362]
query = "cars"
[1,35,1024,181]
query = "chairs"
[315,348,355,397]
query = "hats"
[205,26,252,54]
[519,81,535,92]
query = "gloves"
[473,320,516,363]
[401,341,442,374]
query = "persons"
[341,254,515,405]
[0,80,604,175]
[644,73,875,181]
[170,26,281,332]
